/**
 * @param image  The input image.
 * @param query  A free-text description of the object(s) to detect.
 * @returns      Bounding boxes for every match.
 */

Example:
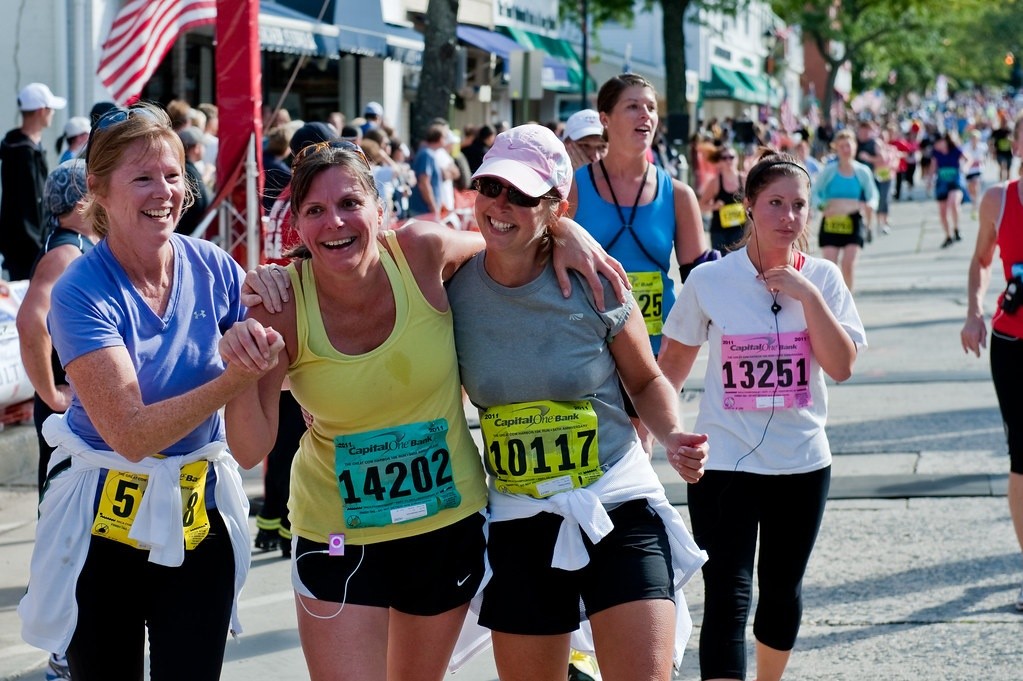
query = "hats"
[364,102,383,115]
[64,116,92,139]
[470,123,573,200]
[18,83,67,111]
[563,109,604,141]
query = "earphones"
[746,206,754,221]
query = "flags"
[95,2,216,107]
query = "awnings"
[704,63,782,109]
[194,0,598,98]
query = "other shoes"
[254,529,279,550]
[279,538,292,558]
[943,238,953,247]
[46,652,72,681]
[955,229,961,241]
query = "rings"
[269,268,281,274]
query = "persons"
[647,90,1023,291]
[218,142,632,681]
[16,159,100,681]
[562,73,708,680]
[961,117,1023,613]
[0,82,610,282]
[237,123,708,681]
[637,151,868,681]
[16,108,285,681]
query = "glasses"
[722,156,735,160]
[478,178,560,208]
[86,108,159,164]
